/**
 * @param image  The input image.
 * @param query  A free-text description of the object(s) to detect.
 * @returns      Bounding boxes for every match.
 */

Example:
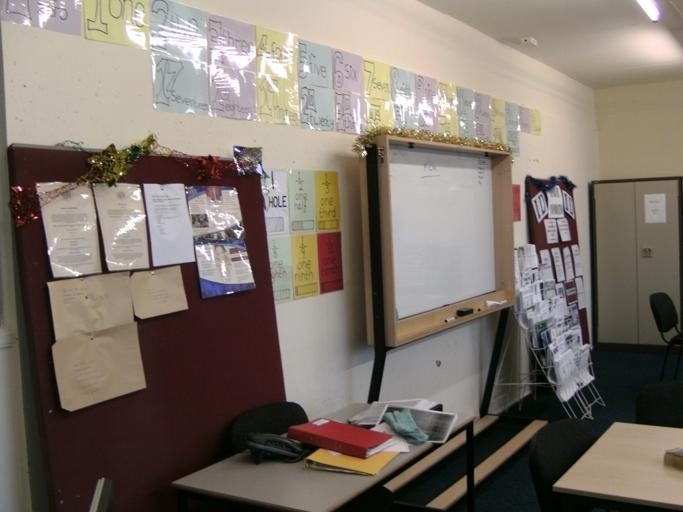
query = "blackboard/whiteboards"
[6,145,293,510]
[357,134,515,348]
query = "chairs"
[635,379,682,429]
[529,419,616,510]
[224,399,394,510]
[650,293,683,380]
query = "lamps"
[636,0,661,23]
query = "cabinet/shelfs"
[591,176,683,354]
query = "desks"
[171,404,478,512]
[553,422,683,512]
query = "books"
[288,416,396,457]
[355,399,427,428]
[514,242,596,406]
[375,404,457,444]
[662,445,683,471]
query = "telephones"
[244,431,306,464]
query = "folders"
[288,417,400,477]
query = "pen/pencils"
[445,317,458,322]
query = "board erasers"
[457,308,473,316]
